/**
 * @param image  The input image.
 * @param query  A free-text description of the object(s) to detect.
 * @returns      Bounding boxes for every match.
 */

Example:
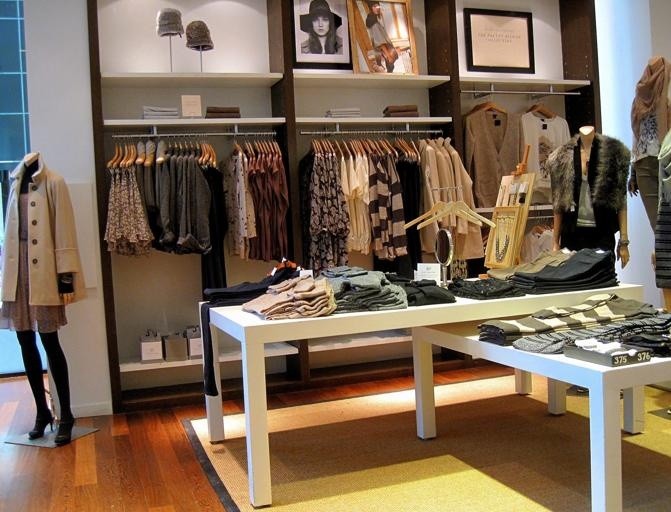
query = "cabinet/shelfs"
[447,1,608,371]
[281,0,464,393]
[84,1,312,416]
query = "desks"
[195,279,647,511]
[408,307,671,511]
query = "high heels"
[29,411,53,438]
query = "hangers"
[301,130,458,165]
[466,93,567,124]
[105,131,284,173]
[403,188,497,231]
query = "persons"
[546,125,632,285]
[625,55,670,237]
[299,0,346,54]
[3,149,86,447]
[652,128,671,318]
[363,0,400,74]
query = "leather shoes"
[54,417,74,443]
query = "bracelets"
[618,237,631,249]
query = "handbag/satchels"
[381,46,398,63]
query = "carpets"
[180,371,671,511]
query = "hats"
[300,0,342,33]
[186,21,213,50]
[157,9,183,37]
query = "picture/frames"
[482,206,522,273]
[281,0,353,74]
[461,7,536,73]
[347,0,420,76]
